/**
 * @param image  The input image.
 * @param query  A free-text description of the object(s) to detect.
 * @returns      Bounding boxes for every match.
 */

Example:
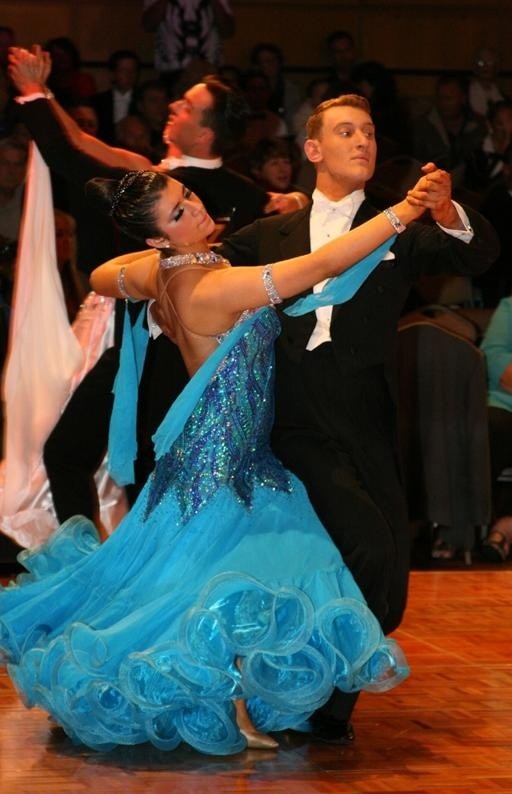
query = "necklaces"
[157,249,232,271]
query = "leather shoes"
[314,726,354,744]
[240,728,279,750]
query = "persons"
[245,137,302,194]
[172,41,300,233]
[2,25,176,325]
[307,32,401,123]
[3,164,446,759]
[8,44,307,531]
[374,65,510,566]
[144,87,505,752]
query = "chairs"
[397,321,491,564]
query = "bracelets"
[383,205,408,235]
[293,195,303,209]
